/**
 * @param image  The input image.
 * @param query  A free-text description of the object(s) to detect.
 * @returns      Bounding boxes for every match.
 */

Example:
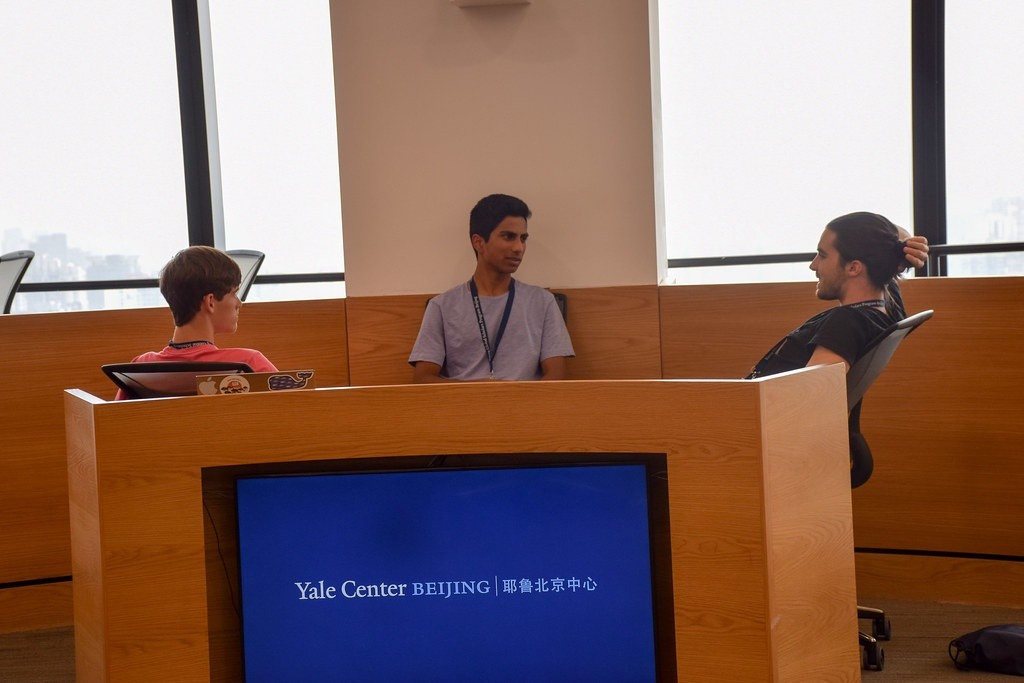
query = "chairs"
[226,250,265,302]
[842,308,935,669]
[101,362,255,399]
[0,250,35,315]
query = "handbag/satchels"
[949,623,1024,677]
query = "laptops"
[196,370,317,396]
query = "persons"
[409,194,575,383]
[115,246,279,402]
[740,212,929,380]
